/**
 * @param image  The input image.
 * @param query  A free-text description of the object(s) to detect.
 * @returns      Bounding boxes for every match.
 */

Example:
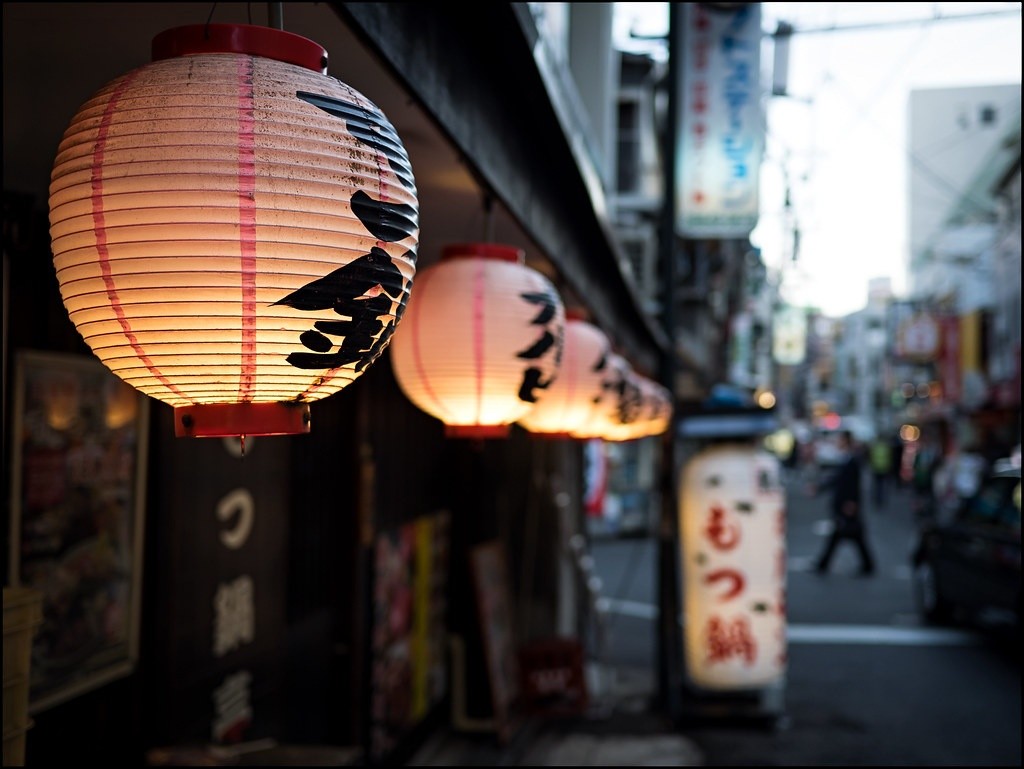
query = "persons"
[810,430,876,576]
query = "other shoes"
[807,565,830,577]
[871,496,881,504]
[856,566,875,576]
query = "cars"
[910,469,1024,655]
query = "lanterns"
[569,352,674,443]
[48,23,419,454]
[390,242,565,437]
[516,309,610,434]
